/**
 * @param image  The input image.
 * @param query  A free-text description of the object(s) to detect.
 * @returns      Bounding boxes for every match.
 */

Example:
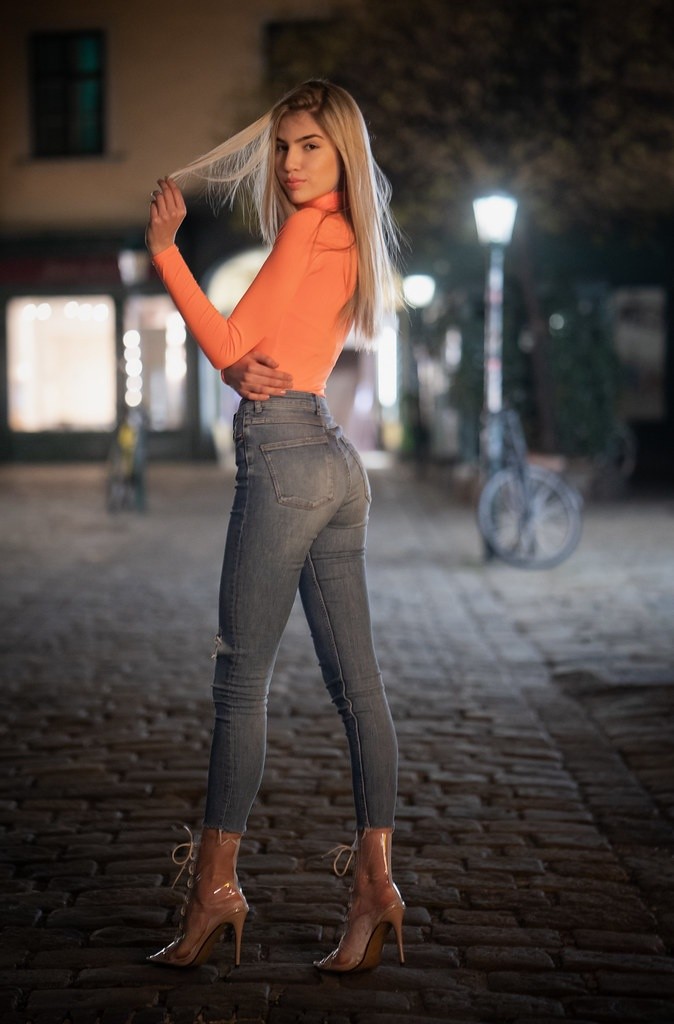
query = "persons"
[145,80,410,980]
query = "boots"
[313,827,406,972]
[144,825,249,966]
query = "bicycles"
[475,398,583,570]
